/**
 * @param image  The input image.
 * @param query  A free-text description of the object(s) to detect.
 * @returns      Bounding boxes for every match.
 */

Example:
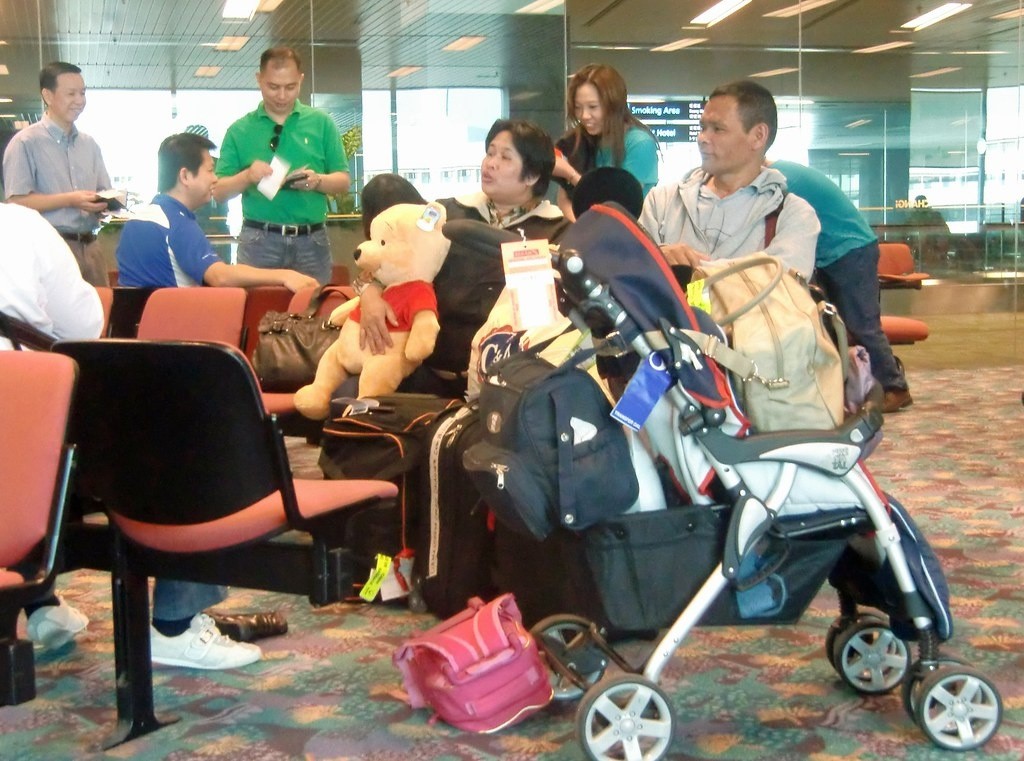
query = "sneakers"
[877,386,913,412]
[27,589,89,650]
[147,611,262,671]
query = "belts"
[242,219,325,238]
[60,230,97,244]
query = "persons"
[552,64,658,225]
[0,201,262,671]
[763,156,913,413]
[639,81,823,295]
[904,196,950,233]
[115,133,320,294]
[212,47,349,290]
[2,60,112,289]
[174,125,231,265]
[361,173,427,241]
[330,119,556,402]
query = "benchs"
[876,243,930,345]
[0,285,399,753]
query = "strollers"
[532,202,1003,761]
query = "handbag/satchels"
[690,252,850,431]
[313,391,462,602]
[248,282,356,393]
[407,395,494,616]
[450,324,640,542]
[389,592,553,734]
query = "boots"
[203,607,289,642]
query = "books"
[91,189,128,210]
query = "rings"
[305,184,309,188]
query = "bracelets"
[314,174,322,191]
[567,171,576,185]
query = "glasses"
[269,125,282,152]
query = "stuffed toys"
[294,202,452,419]
